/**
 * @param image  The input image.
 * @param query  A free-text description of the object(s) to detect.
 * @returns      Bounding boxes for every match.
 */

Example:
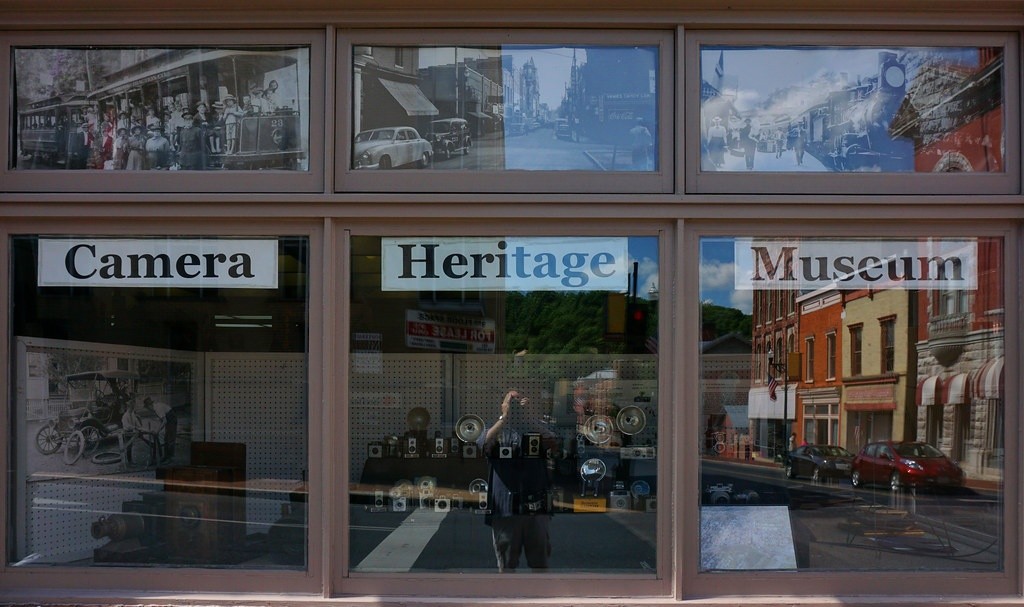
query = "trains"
[760,51,914,172]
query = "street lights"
[767,348,788,453]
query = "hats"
[223,93,237,104]
[119,111,129,117]
[193,100,208,110]
[744,118,751,122]
[254,87,263,94]
[143,397,155,409]
[211,101,227,108]
[150,125,164,131]
[180,112,194,118]
[117,127,129,136]
[712,117,722,123]
[125,399,135,409]
[181,105,193,111]
[131,125,144,134]
[797,120,804,124]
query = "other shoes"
[225,150,233,156]
[211,147,222,153]
[170,144,176,152]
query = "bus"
[20,92,102,164]
[86,47,306,170]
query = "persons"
[143,397,177,465]
[523,123,529,136]
[727,126,798,159]
[800,438,808,446]
[629,117,653,171]
[707,116,727,167]
[789,432,797,451]
[122,400,142,465]
[740,119,758,169]
[118,380,129,400]
[78,80,278,169]
[792,120,807,165]
[476,388,562,572]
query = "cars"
[353,126,435,170]
[850,440,964,495]
[508,117,573,140]
[425,117,472,160]
[783,444,857,482]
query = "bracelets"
[135,426,136,429]
[499,416,505,420]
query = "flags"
[768,373,778,400]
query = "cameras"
[510,399,524,414]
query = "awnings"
[941,373,971,404]
[971,355,1005,398]
[376,77,440,116]
[916,376,942,406]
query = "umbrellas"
[194,49,297,151]
